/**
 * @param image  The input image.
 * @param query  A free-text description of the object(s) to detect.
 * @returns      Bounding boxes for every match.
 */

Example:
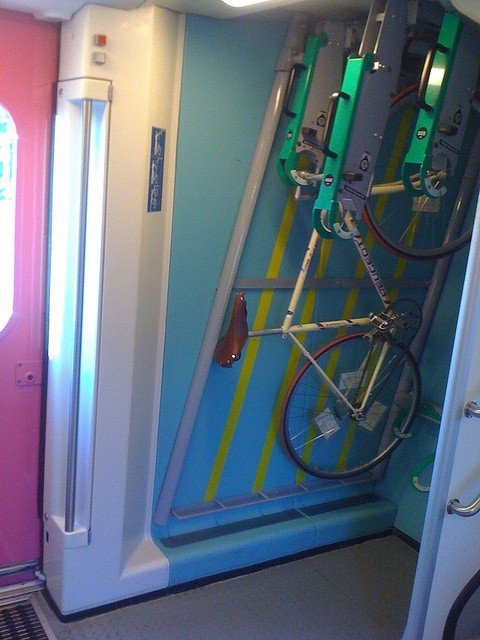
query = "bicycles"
[212,82,479,483]
